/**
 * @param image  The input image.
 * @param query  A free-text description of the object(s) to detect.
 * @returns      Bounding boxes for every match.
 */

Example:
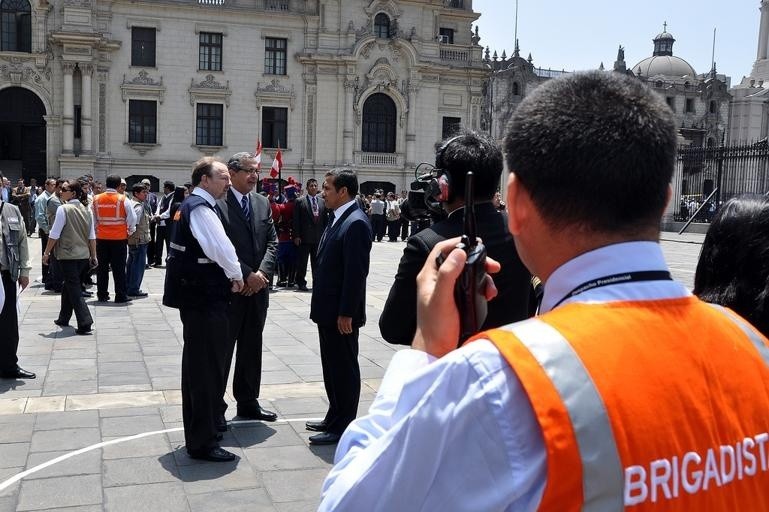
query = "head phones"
[428,134,468,195]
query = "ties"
[312,197,319,221]
[241,196,250,221]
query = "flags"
[270,146,283,179]
[251,142,265,174]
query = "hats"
[121,179,126,184]
[142,179,150,185]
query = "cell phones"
[499,202,505,210]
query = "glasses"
[240,168,261,174]
[62,188,72,192]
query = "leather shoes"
[187,447,234,462]
[237,407,276,420]
[0,367,36,379]
[306,422,341,445]
[115,290,148,303]
[54,319,69,326]
[75,326,91,334]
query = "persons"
[162,155,245,462]
[302,168,374,445]
[317,70,768,512]
[378,129,537,345]
[676,195,725,223]
[218,152,279,431]
[691,192,769,343]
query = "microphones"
[424,193,446,215]
